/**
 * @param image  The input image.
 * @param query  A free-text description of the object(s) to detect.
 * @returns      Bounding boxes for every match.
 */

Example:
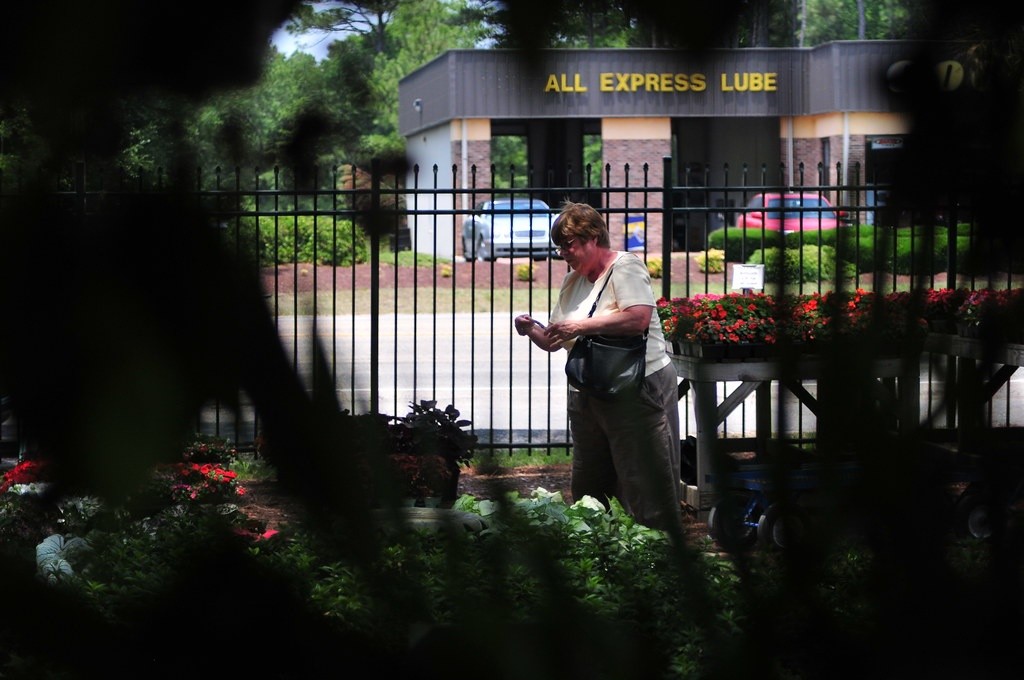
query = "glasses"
[555,235,581,256]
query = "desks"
[923,332,1024,458]
[666,346,918,552]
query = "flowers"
[0,434,280,558]
[656,288,1024,342]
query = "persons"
[515,197,681,549]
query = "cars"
[462,198,565,262]
[737,193,849,232]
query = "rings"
[520,327,523,331]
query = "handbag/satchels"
[565,267,648,403]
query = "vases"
[668,320,1024,359]
